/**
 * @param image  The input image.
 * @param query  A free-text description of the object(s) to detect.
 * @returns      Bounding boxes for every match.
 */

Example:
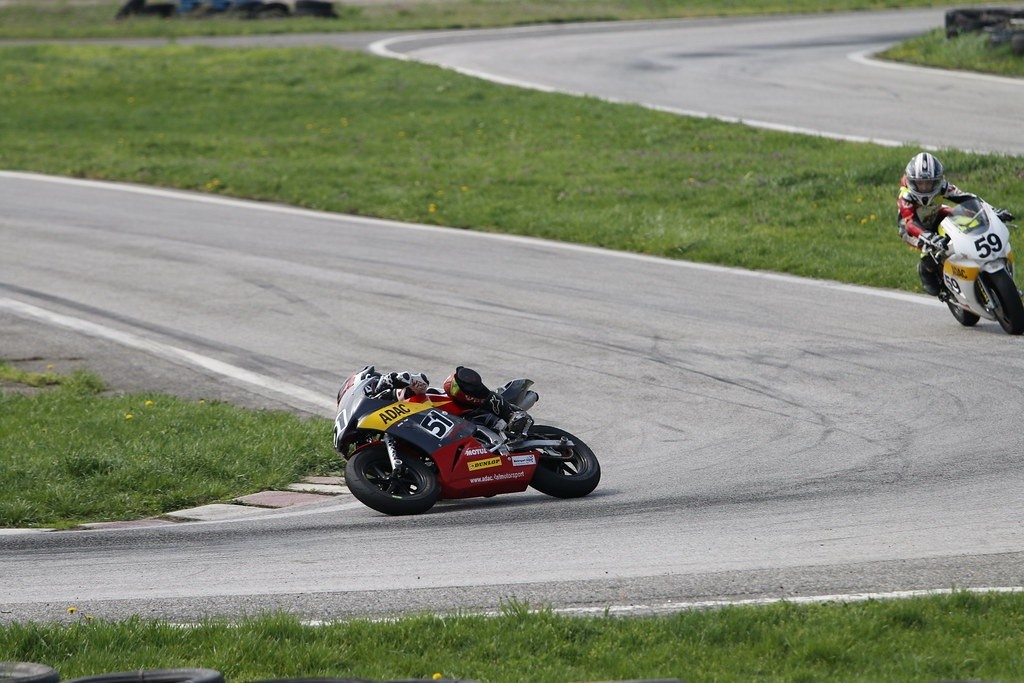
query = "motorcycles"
[333,376,601,516]
[928,197,1024,335]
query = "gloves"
[373,370,401,393]
[920,231,949,252]
[987,202,1016,223]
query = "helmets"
[336,365,380,402]
[905,152,948,207]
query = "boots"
[916,254,941,297]
[483,391,535,434]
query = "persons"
[899,155,1013,294]
[338,366,535,456]
